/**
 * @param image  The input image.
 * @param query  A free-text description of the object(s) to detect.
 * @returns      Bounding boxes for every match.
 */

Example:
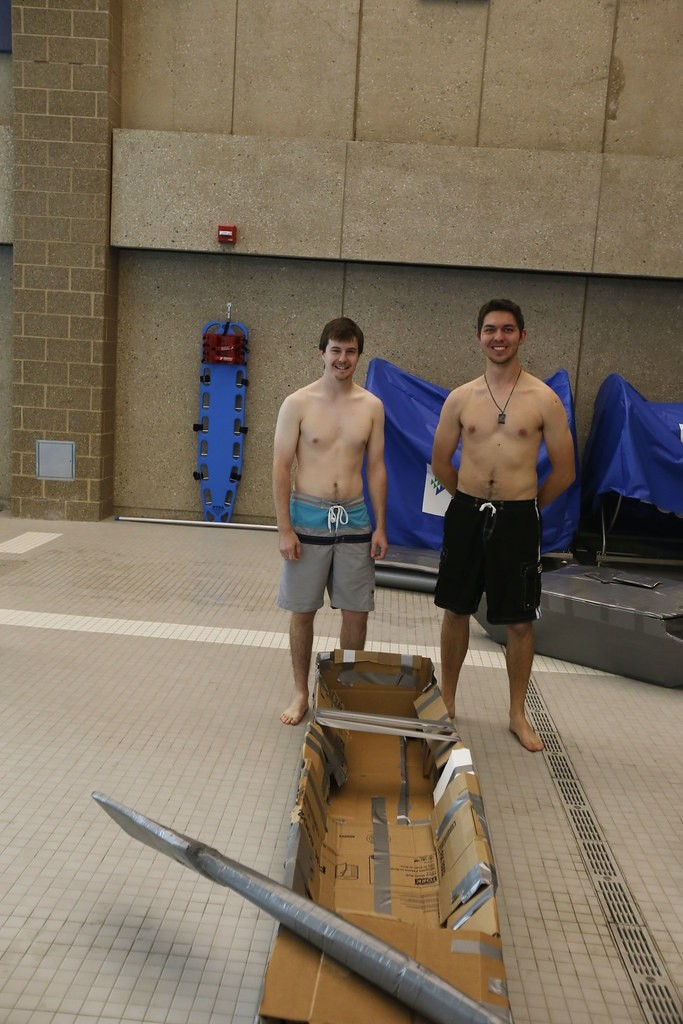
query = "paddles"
[91,790,508,1024]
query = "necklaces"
[484,367,523,426]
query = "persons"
[432,298,575,752]
[272,318,388,725]
